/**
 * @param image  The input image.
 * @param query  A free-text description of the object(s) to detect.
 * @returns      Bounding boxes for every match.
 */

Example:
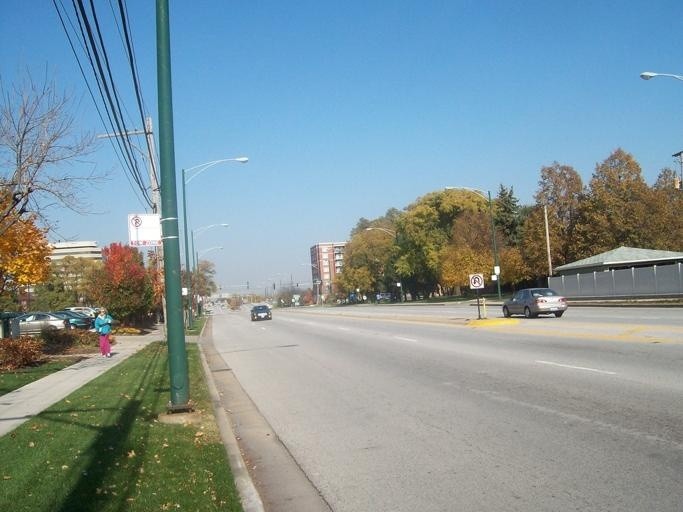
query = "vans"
[379,292,396,300]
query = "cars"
[248,304,273,322]
[0,300,119,340]
[501,287,568,319]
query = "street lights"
[365,225,407,303]
[180,155,249,333]
[444,183,504,303]
[301,260,320,306]
[639,68,682,83]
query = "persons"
[376,293,381,303]
[95,307,112,358]
